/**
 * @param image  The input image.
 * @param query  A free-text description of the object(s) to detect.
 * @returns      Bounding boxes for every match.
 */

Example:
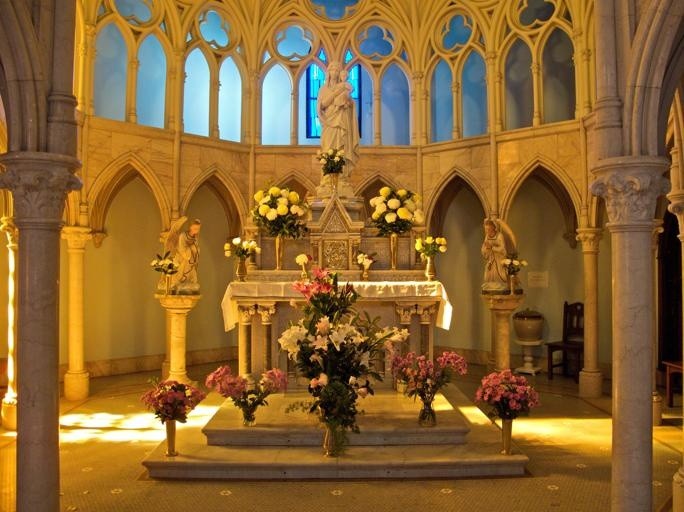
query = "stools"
[662,359,683,411]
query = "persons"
[332,70,352,113]
[170,219,203,294]
[479,218,512,284]
[314,60,362,183]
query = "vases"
[323,425,340,458]
[163,273,175,297]
[300,266,306,280]
[417,400,437,426]
[390,231,399,270]
[425,256,436,281]
[508,275,517,295]
[165,419,177,456]
[363,267,369,281]
[329,172,339,194]
[501,420,513,454]
[241,407,257,427]
[236,257,247,282]
[275,235,285,271]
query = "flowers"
[143,379,204,423]
[224,237,261,258]
[318,148,349,175]
[250,187,308,240]
[369,186,424,234]
[151,251,181,273]
[296,254,311,266]
[475,368,536,419]
[391,350,468,399]
[204,366,288,410]
[356,250,378,267]
[501,257,527,274]
[278,269,409,426]
[415,235,447,258]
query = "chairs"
[544,297,586,383]
